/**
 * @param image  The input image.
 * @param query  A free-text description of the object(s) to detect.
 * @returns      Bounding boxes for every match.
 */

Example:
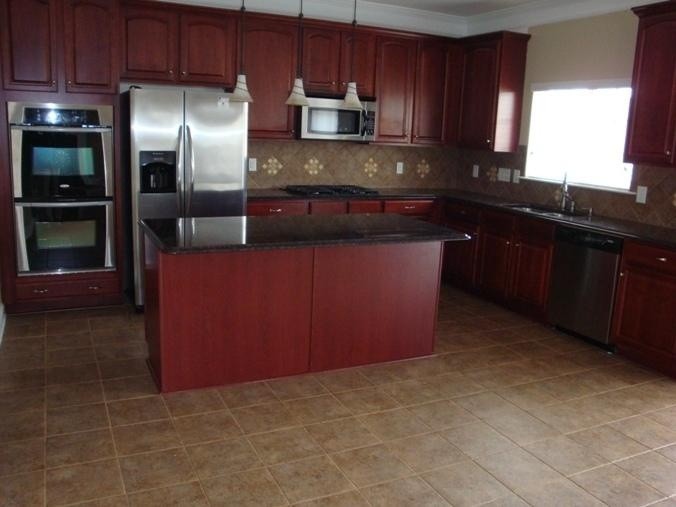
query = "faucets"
[559,184,568,210]
[564,193,575,212]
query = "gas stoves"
[286,185,378,196]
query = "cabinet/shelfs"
[458,30,532,154]
[237,12,459,149]
[609,237,676,379]
[0,0,237,105]
[623,0,676,169]
[0,272,123,315]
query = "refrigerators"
[119,85,249,309]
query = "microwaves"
[297,97,377,143]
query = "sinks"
[513,206,549,213]
[542,213,569,219]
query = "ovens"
[7,101,117,277]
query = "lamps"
[285,0,309,106]
[230,0,255,103]
[340,1,364,109]
